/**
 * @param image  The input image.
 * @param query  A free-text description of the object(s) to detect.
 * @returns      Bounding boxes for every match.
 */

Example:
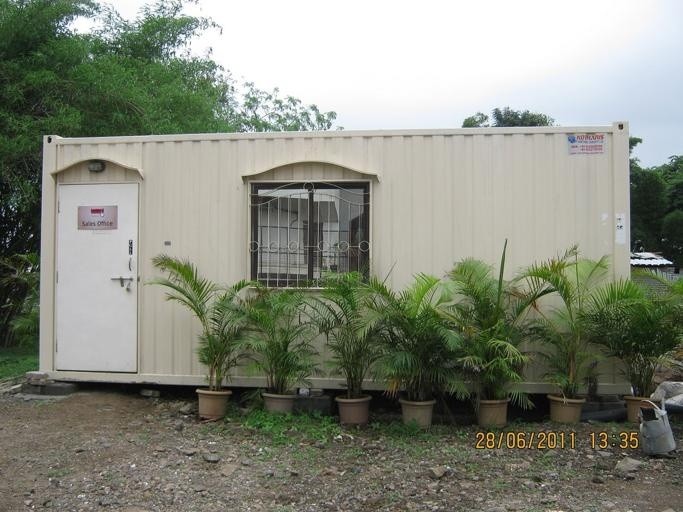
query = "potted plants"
[432,255,542,427]
[508,244,606,427]
[145,243,260,428]
[209,272,330,424]
[356,271,451,430]
[295,260,386,428]
[548,267,681,427]
